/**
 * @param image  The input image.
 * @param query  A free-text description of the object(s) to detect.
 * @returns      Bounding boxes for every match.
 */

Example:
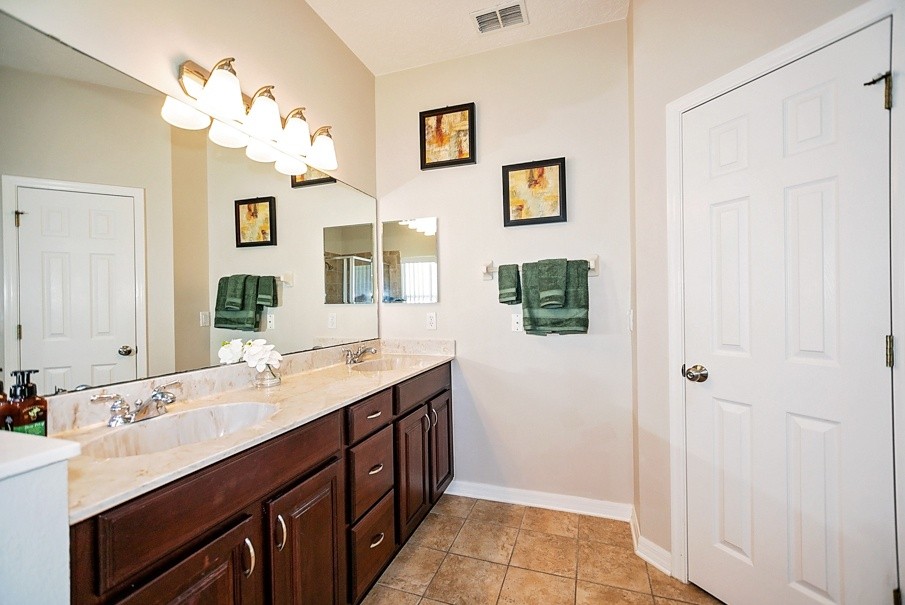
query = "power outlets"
[425,311,438,332]
[328,311,338,330]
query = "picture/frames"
[417,101,478,172]
[290,165,337,188]
[232,195,279,249]
[501,156,568,229]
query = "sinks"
[78,400,280,460]
[350,357,421,372]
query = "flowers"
[240,337,284,378]
[218,338,244,365]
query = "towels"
[538,258,568,308]
[225,274,251,311]
[498,264,522,305]
[521,259,590,337]
[256,276,278,307]
[214,275,263,333]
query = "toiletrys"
[9,369,48,437]
[8,369,26,403]
[0,367,11,429]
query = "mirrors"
[0,8,381,404]
[381,215,441,307]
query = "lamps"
[160,95,212,132]
[177,54,340,172]
[274,150,307,176]
[245,138,278,164]
[208,118,250,149]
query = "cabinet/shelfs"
[68,361,455,605]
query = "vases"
[252,361,282,390]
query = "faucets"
[343,344,377,365]
[91,379,184,427]
